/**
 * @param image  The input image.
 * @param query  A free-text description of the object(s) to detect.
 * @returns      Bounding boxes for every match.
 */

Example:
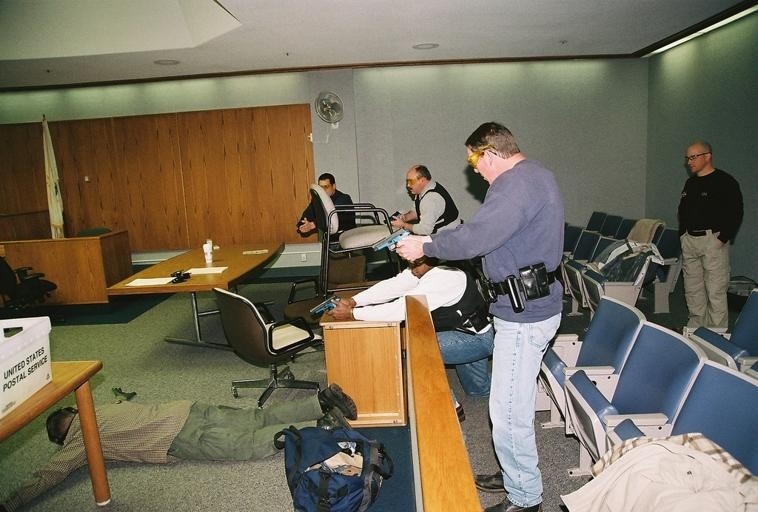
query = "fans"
[314,90,345,129]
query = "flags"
[41,119,67,239]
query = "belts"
[684,229,722,236]
[482,269,558,295]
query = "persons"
[389,121,564,512]
[296,173,356,242]
[677,141,744,328]
[390,165,461,236]
[327,256,494,422]
[0,383,357,511]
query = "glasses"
[319,183,334,190]
[684,152,712,161]
[404,178,420,186]
[465,142,494,169]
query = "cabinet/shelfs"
[319,282,408,429]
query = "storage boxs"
[0,314,54,421]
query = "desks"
[106,242,286,350]
[0,360,112,506]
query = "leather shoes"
[455,403,465,423]
[318,382,358,421]
[482,496,542,512]
[473,469,506,493]
[317,405,353,431]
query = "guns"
[374,228,421,262]
[296,217,304,226]
[111,385,137,402]
[384,212,403,226]
[308,294,339,316]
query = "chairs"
[309,184,400,297]
[561,320,707,476]
[0,256,58,316]
[210,287,323,409]
[608,359,757,475]
[682,288,758,371]
[536,295,647,428]
[559,210,684,331]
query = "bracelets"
[350,307,355,320]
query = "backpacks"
[273,426,395,512]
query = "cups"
[202,244,212,262]
